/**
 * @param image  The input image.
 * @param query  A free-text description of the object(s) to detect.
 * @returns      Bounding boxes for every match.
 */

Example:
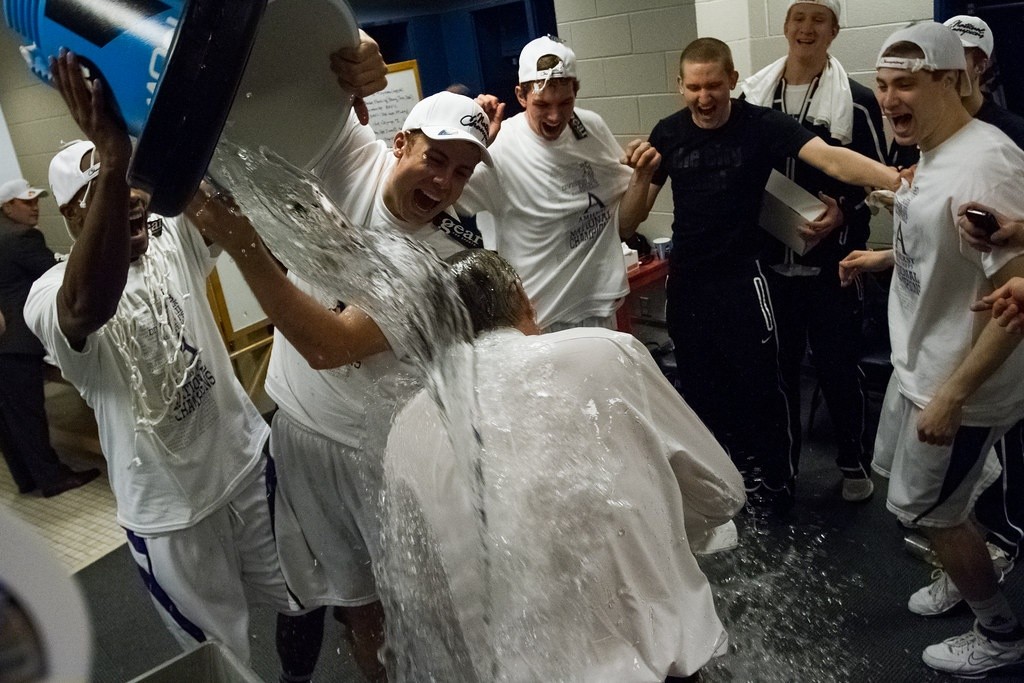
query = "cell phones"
[965,208,1009,247]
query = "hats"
[0,178,48,207]
[402,90,495,169]
[876,21,972,98]
[518,36,579,93]
[49,139,98,206]
[784,0,839,24]
[943,15,994,58]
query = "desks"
[614,253,670,334]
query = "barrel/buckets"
[0,0,362,218]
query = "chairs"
[805,271,890,444]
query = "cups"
[653,238,672,261]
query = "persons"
[181,30,495,683]
[0,180,101,499]
[737,0,888,501]
[386,248,747,683]
[641,37,917,526]
[838,16,1024,679]
[456,35,662,334]
[23,47,327,683]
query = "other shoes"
[985,541,1015,575]
[734,451,764,493]
[833,456,874,501]
[17,482,45,496]
[761,460,793,492]
[45,467,102,496]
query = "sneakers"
[922,620,1024,674]
[909,557,1005,614]
